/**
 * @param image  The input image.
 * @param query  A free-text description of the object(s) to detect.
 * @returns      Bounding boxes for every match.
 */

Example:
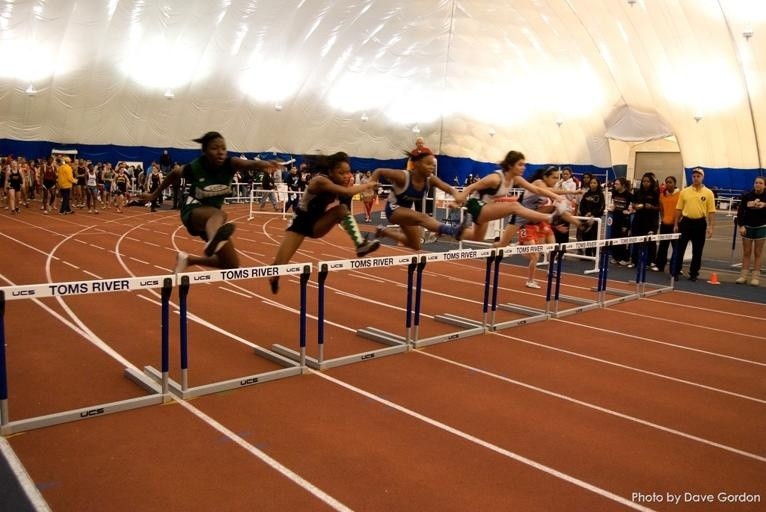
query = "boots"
[750,270,761,287]
[735,270,749,285]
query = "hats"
[691,168,704,177]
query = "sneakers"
[650,267,661,272]
[203,222,235,258]
[649,262,656,267]
[627,264,635,268]
[551,205,563,226]
[609,259,617,265]
[454,217,468,242]
[690,275,698,282]
[356,239,380,258]
[678,270,684,275]
[526,281,541,289]
[618,259,631,266]
[270,278,279,296]
[172,251,187,275]
[672,276,679,282]
[373,224,385,241]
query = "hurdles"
[142,260,314,400]
[365,247,499,364]
[591,232,685,299]
[270,252,419,371]
[506,238,610,320]
[555,233,648,309]
[247,189,303,222]
[223,182,264,205]
[0,274,177,437]
[442,241,560,359]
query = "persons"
[453,176,459,186]
[490,165,716,289]
[135,131,283,274]
[736,175,766,286]
[473,175,480,183]
[429,151,565,243]
[407,136,433,171]
[467,176,473,185]
[465,174,473,184]
[267,152,381,294]
[369,151,467,251]
[0,156,379,222]
